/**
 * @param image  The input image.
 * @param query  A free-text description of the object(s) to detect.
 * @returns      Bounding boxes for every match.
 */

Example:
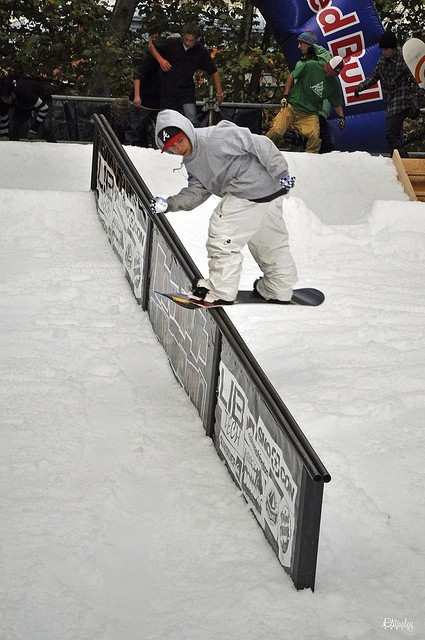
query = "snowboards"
[400,38,424,91]
[154,288,325,309]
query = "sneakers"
[187,278,234,304]
[254,278,291,304]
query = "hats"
[324,55,344,77]
[297,30,318,45]
[378,30,397,49]
[158,127,184,155]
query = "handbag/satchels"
[408,99,420,120]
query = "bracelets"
[337,115,345,119]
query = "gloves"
[149,196,168,215]
[280,94,289,108]
[336,115,346,129]
[279,175,295,191]
[354,84,363,98]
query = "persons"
[150,109,298,303]
[354,30,420,158]
[123,49,163,149]
[298,32,335,154]
[146,20,223,128]
[265,55,346,153]
[216,93,223,97]
[0,75,56,143]
